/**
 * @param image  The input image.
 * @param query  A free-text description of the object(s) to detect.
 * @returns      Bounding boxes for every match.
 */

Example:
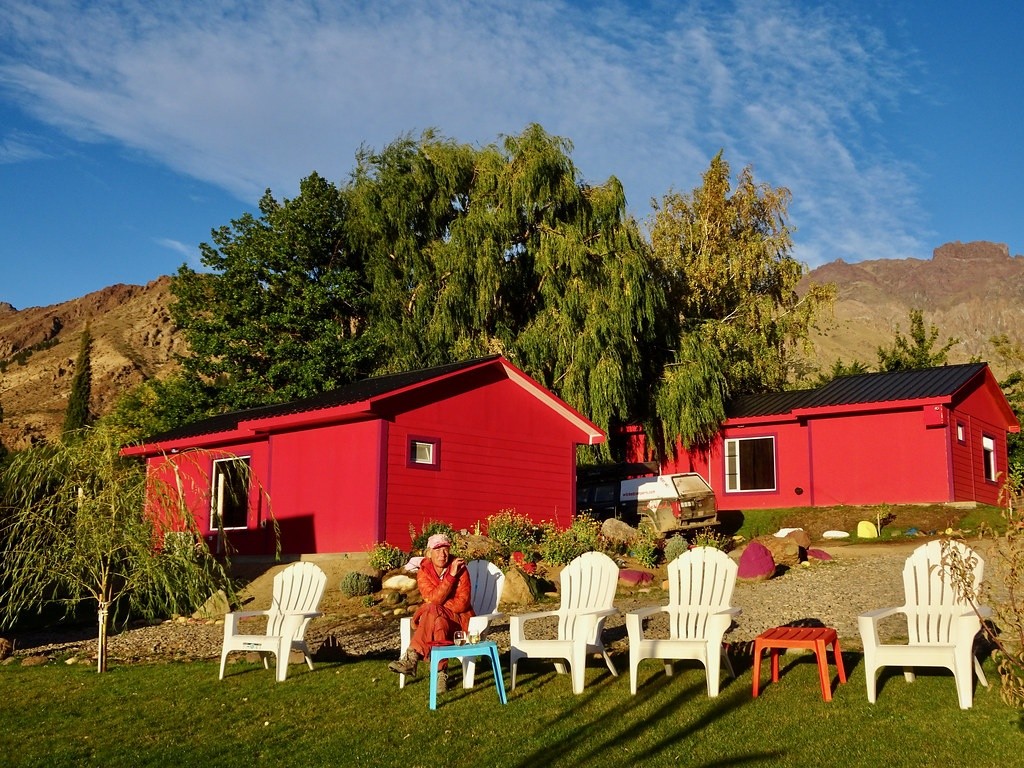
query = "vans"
[575,472,723,538]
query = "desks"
[752,627,847,702]
[429,641,507,710]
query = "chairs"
[399,560,505,689]
[219,561,328,682]
[509,552,620,694]
[626,547,743,698]
[858,540,989,710]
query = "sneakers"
[436,670,450,695]
[387,647,420,678]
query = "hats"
[426,533,453,549]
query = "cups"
[468,630,480,645]
[454,631,466,647]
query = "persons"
[388,533,477,696]
